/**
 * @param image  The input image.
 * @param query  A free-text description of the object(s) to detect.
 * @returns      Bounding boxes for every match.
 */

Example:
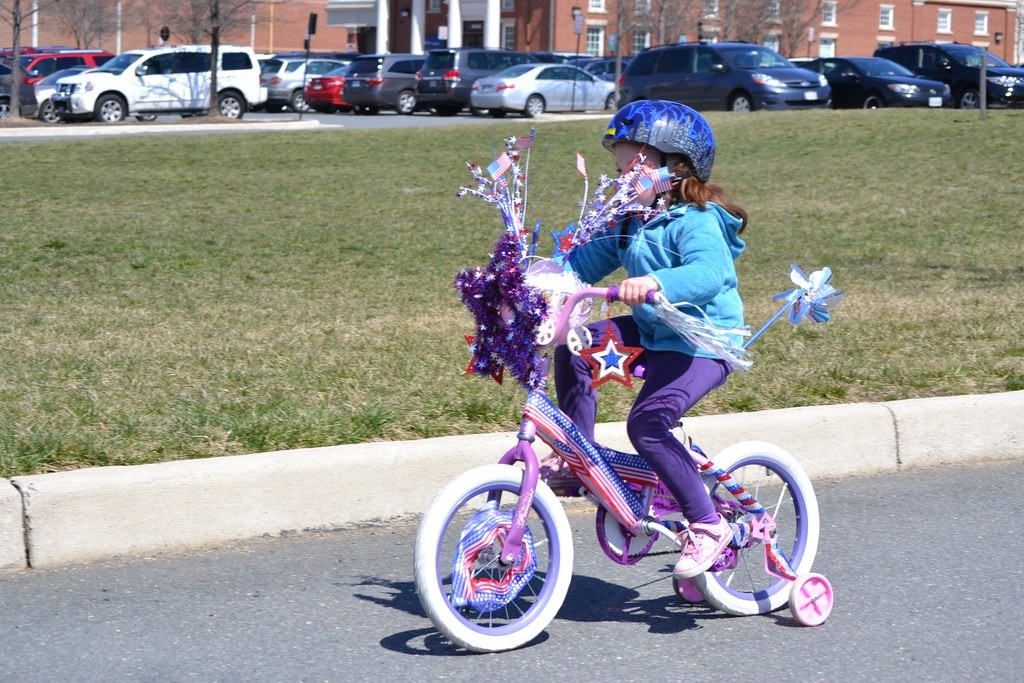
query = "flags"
[485,132,532,191]
[575,152,588,179]
[621,163,672,196]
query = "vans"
[256,51,362,69]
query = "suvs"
[619,39,833,114]
[12,49,118,85]
[874,40,1024,110]
[1,47,80,57]
[415,48,558,116]
[339,52,477,115]
[50,43,268,122]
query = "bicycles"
[413,285,832,653]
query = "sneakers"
[537,450,581,487]
[672,512,733,579]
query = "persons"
[539,98,748,579]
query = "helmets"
[602,98,716,183]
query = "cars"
[260,57,349,113]
[0,60,37,119]
[470,62,621,117]
[532,52,633,81]
[777,59,851,76]
[785,57,952,109]
[26,66,157,121]
[302,61,360,114]
